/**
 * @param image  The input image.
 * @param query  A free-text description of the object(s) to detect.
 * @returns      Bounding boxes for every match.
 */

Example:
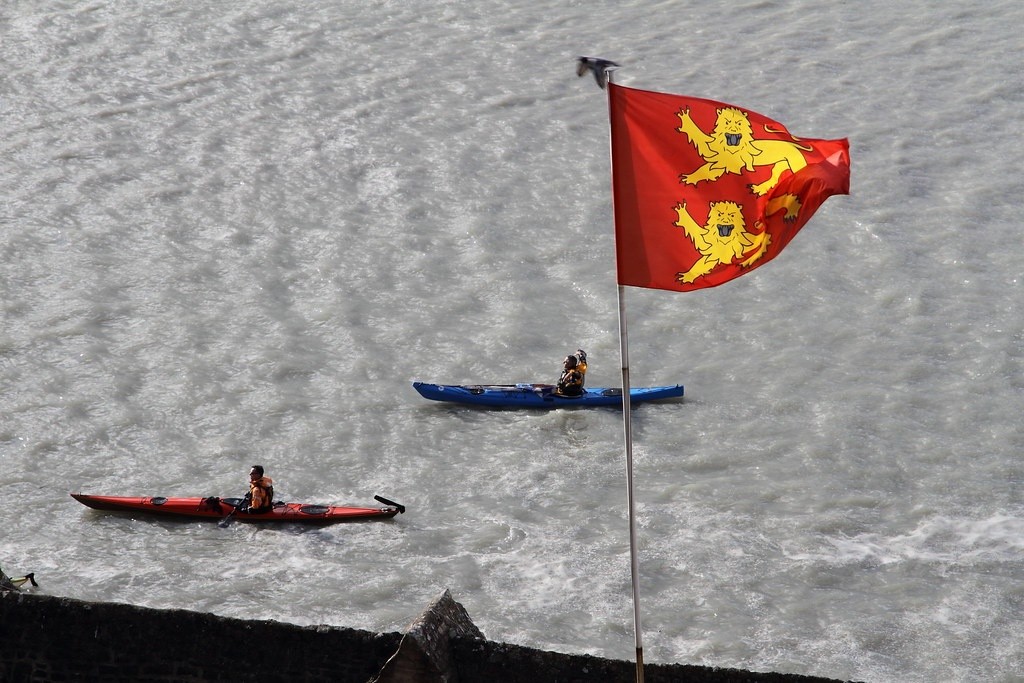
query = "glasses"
[251,472,258,475]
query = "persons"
[553,350,587,396]
[241,465,273,514]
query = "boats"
[68,493,406,524]
[411,381,685,409]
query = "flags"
[607,82,851,291]
